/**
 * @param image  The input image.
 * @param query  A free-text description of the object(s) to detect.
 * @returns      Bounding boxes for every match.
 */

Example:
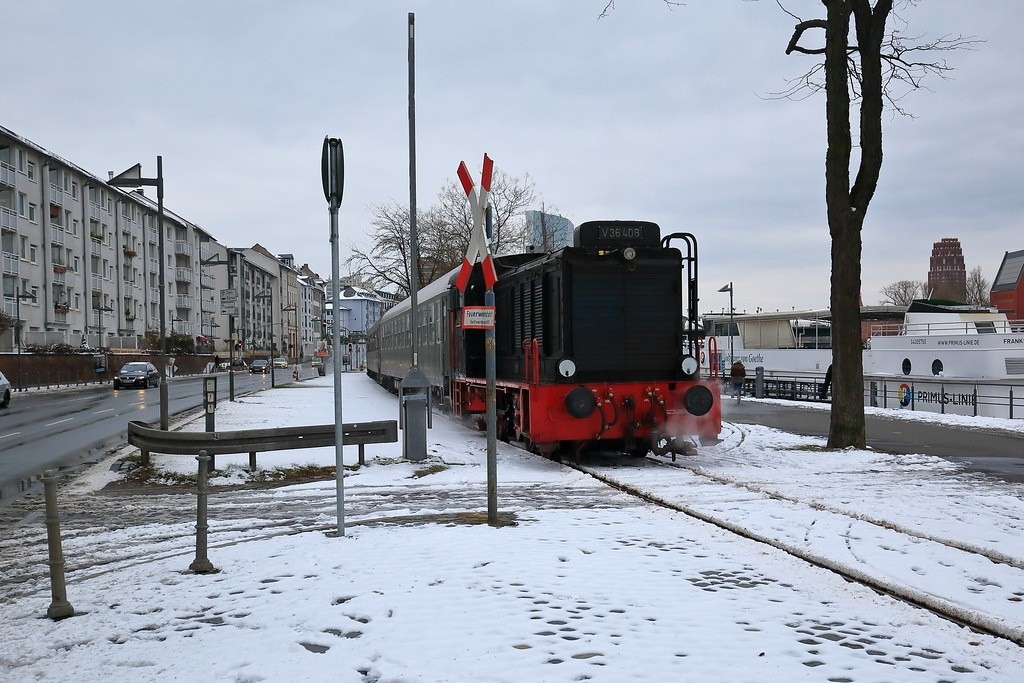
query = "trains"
[366,220,722,460]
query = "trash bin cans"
[402,393,427,461]
[318,363,326,376]
[755,366,765,397]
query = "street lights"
[106,155,169,435]
[204,252,234,404]
[717,282,734,367]
[254,286,275,389]
[280,301,298,366]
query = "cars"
[250,359,270,374]
[0,371,11,408]
[219,358,247,371]
[274,357,288,369]
[310,358,322,366]
[113,361,160,389]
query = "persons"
[819,364,832,399]
[731,360,746,404]
[214,354,219,368]
[318,342,326,352]
